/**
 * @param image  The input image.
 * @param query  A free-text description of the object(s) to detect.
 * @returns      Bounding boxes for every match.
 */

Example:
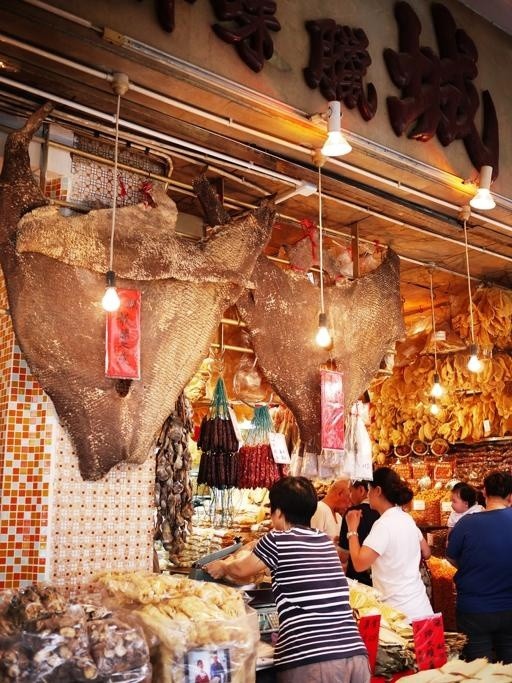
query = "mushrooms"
[0,585,154,683]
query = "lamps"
[101,73,129,311]
[463,166,496,211]
[314,146,333,346]
[457,206,482,374]
[427,261,444,398]
[312,101,352,159]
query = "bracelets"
[346,530,360,542]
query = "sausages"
[196,415,288,490]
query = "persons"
[199,472,377,682]
[345,465,436,675]
[208,649,227,682]
[339,478,381,586]
[445,480,485,549]
[195,660,210,682]
[445,466,511,664]
[309,476,353,541]
